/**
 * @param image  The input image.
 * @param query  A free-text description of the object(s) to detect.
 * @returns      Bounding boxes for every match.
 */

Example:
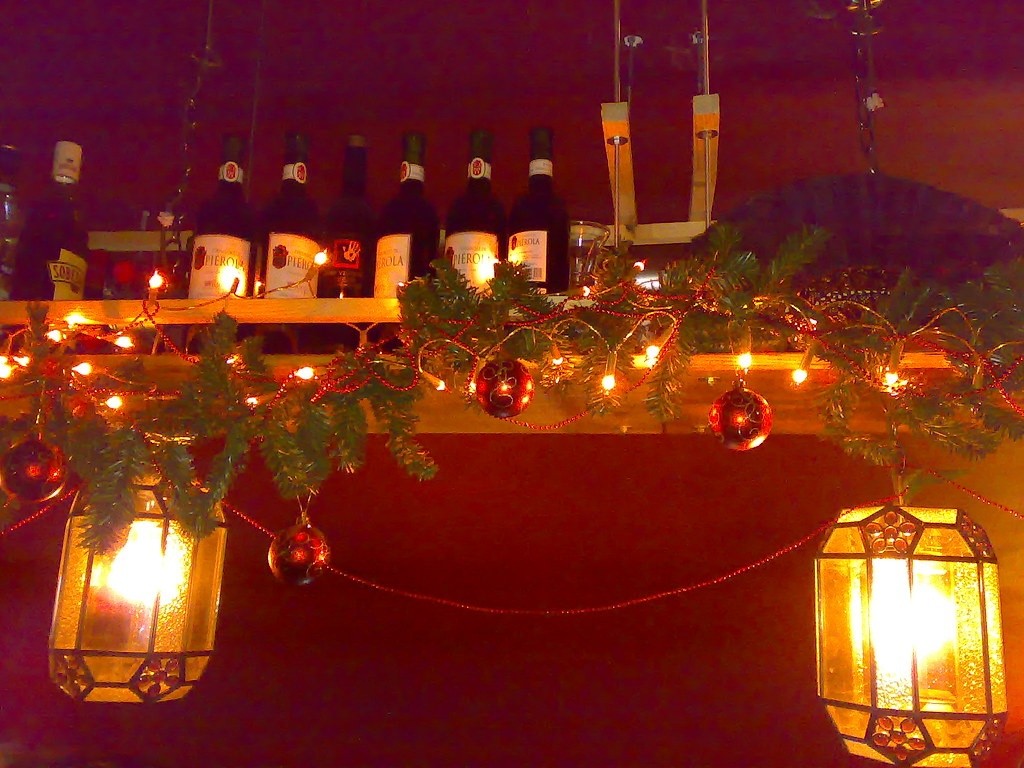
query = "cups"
[568,220,611,299]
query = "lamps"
[813,504,1010,768]
[47,471,230,704]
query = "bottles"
[8,139,90,301]
[445,130,506,300]
[503,126,570,302]
[0,143,25,301]
[320,134,374,298]
[186,133,257,299]
[252,130,323,299]
[374,132,441,299]
